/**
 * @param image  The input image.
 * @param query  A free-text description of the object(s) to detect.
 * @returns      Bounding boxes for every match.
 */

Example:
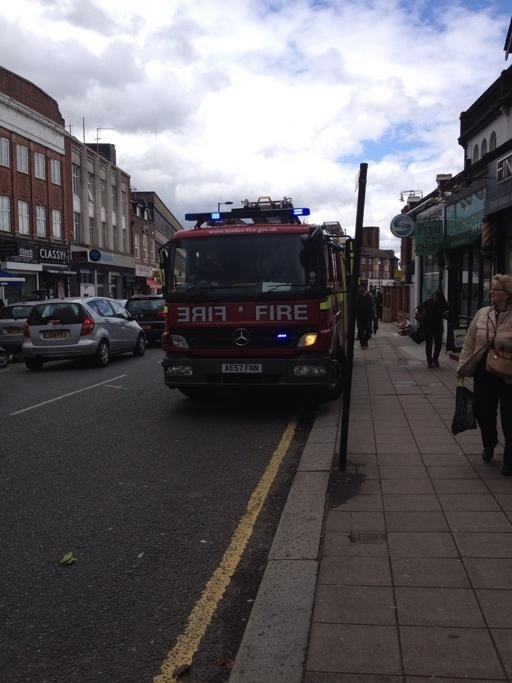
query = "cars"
[0,293,169,371]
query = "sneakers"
[502,464,512,476]
[483,440,498,461]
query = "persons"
[352,280,382,349]
[456,272,511,479]
[413,288,454,370]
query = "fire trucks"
[156,195,356,409]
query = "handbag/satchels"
[485,349,512,378]
[451,386,477,435]
